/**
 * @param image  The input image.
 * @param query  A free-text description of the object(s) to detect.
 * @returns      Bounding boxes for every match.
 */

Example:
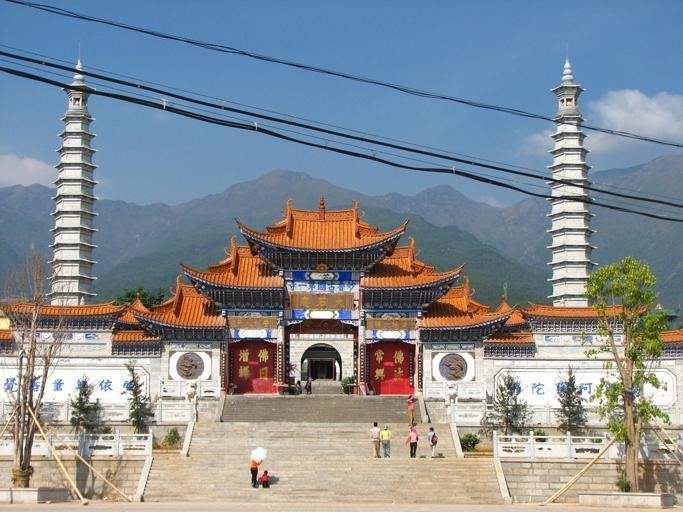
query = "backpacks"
[431,433,438,444]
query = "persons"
[406,394,417,427]
[304,375,312,393]
[427,426,437,457]
[379,424,391,457]
[404,427,418,458]
[249,445,266,488]
[258,469,270,488]
[369,421,381,458]
